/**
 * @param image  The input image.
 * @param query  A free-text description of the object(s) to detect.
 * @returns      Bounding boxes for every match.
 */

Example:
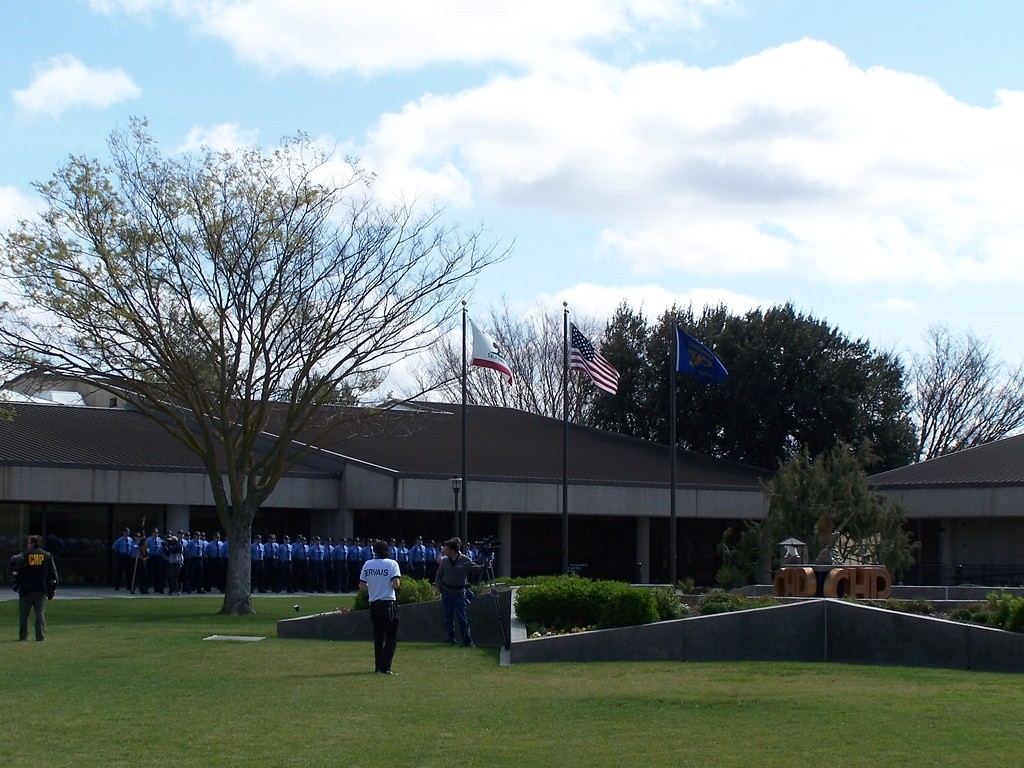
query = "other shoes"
[447,641,475,647]
[115,587,358,594]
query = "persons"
[434,538,495,649]
[251,534,296,594]
[292,533,379,593]
[10,535,59,643]
[359,540,402,677]
[112,527,152,595]
[208,532,231,594]
[146,527,208,596]
[388,535,433,586]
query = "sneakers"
[377,670,399,676]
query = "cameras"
[474,535,501,559]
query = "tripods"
[461,559,510,650]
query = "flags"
[469,316,513,387]
[569,320,621,396]
[674,324,728,386]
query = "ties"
[124,539,437,560]
